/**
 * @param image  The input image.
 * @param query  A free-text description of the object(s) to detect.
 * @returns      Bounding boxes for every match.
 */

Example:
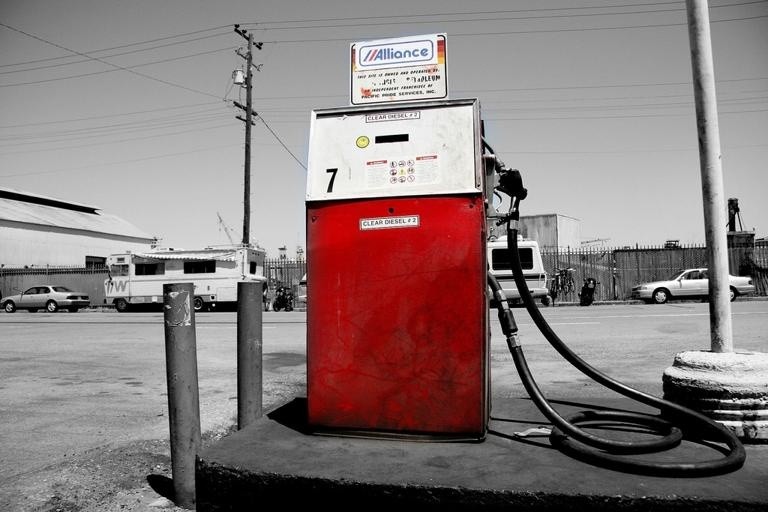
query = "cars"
[631,267,754,304]
[0,285,90,312]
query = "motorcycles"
[578,278,599,306]
[273,286,294,312]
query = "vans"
[487,234,548,302]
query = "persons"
[276,286,291,311]
[263,280,269,311]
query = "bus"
[104,248,265,312]
